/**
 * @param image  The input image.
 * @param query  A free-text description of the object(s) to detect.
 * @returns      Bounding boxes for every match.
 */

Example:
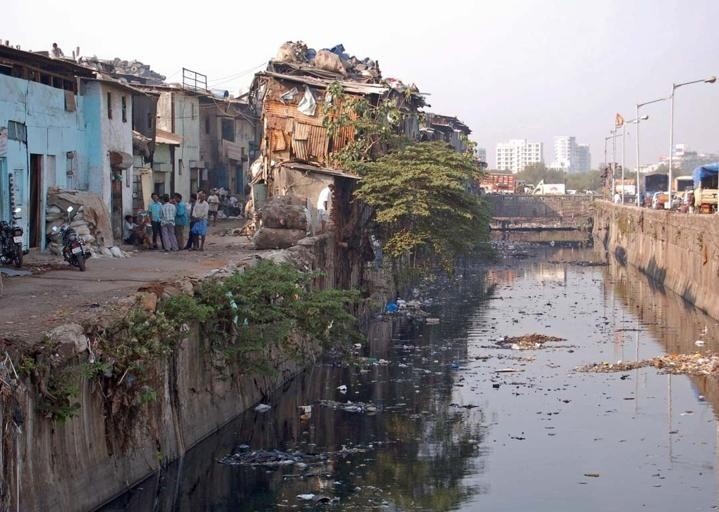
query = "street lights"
[636,97,671,207]
[668,75,716,209]
[604,114,648,207]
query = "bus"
[673,175,693,199]
[693,163,718,209]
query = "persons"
[122,187,244,253]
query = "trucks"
[532,179,566,195]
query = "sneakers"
[145,244,204,251]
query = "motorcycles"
[0,216,29,268]
[47,206,92,272]
